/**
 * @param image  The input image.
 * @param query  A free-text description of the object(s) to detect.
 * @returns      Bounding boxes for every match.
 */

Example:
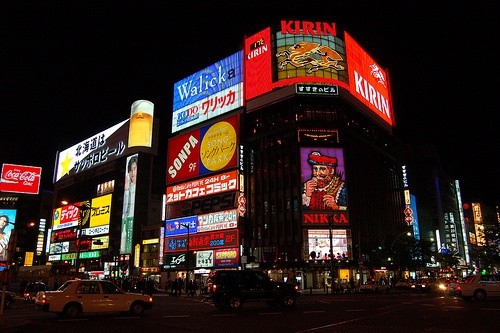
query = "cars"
[360,281,390,294]
[396,277,462,294]
[447,274,500,300]
[0,291,17,308]
[34,279,154,317]
[24,281,47,303]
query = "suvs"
[205,270,301,312]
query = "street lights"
[167,223,190,293]
[62,200,83,278]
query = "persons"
[171,278,204,297]
[331,274,418,295]
[0,215,9,261]
[19,277,28,289]
[54,277,63,291]
[121,155,138,219]
[104,277,155,297]
[321,277,326,292]
